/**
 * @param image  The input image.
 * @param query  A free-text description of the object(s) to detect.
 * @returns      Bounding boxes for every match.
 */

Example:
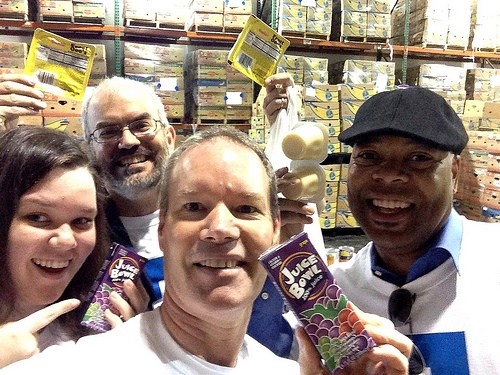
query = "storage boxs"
[268,2,395,228]
[1,1,110,143]
[121,1,255,152]
[397,0,500,225]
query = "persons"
[0,76,295,359]
[0,126,150,370]
[275,86,500,375]
[0,125,414,375]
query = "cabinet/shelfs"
[2,0,500,229]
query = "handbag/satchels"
[264,84,328,266]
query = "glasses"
[388,287,426,375]
[87,118,166,145]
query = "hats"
[338,86,469,156]
